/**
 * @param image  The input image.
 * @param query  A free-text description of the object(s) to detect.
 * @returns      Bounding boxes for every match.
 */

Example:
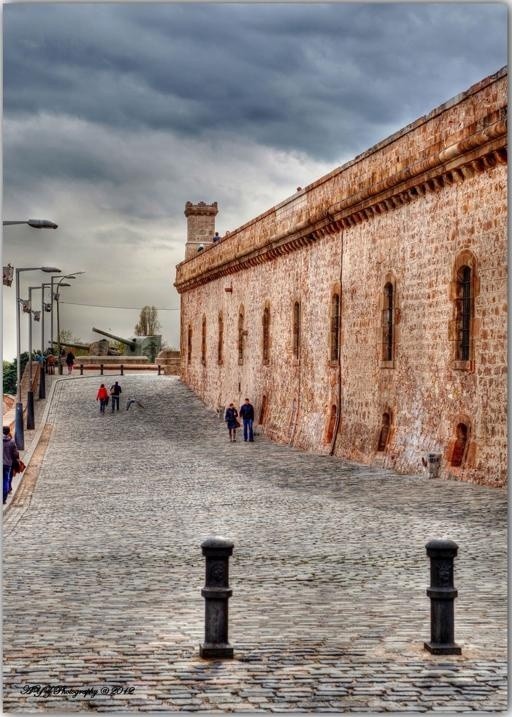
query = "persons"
[225,403,239,442]
[3,425,19,503]
[240,399,255,442]
[34,353,42,363]
[110,381,122,412]
[197,244,204,252]
[46,351,56,375]
[66,351,75,375]
[96,384,109,413]
[213,232,221,243]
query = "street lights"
[28,270,87,429]
[16,266,60,449]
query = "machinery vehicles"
[48,327,162,363]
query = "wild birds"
[126,398,144,411]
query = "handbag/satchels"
[111,387,115,394]
[236,421,240,427]
[106,396,109,406]
[11,458,27,476]
